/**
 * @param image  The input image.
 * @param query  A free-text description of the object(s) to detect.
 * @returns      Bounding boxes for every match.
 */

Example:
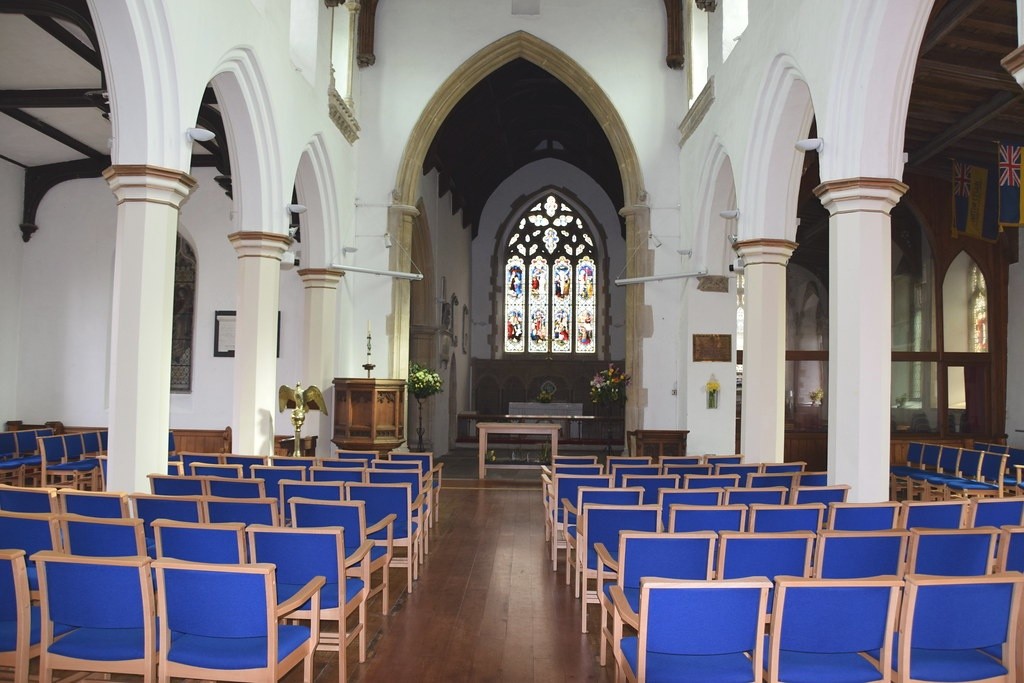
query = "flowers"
[811,388,824,399]
[590,363,633,407]
[408,360,444,398]
[707,381,720,408]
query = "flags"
[950,161,999,243]
[998,141,1024,231]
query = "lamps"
[794,138,825,152]
[719,210,739,220]
[189,127,216,142]
[345,247,358,253]
[676,249,692,257]
[290,205,307,215]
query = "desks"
[477,422,562,479]
[510,401,582,439]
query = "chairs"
[911,413,931,433]
[0,428,442,683]
[542,441,1024,683]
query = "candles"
[368,320,371,332]
